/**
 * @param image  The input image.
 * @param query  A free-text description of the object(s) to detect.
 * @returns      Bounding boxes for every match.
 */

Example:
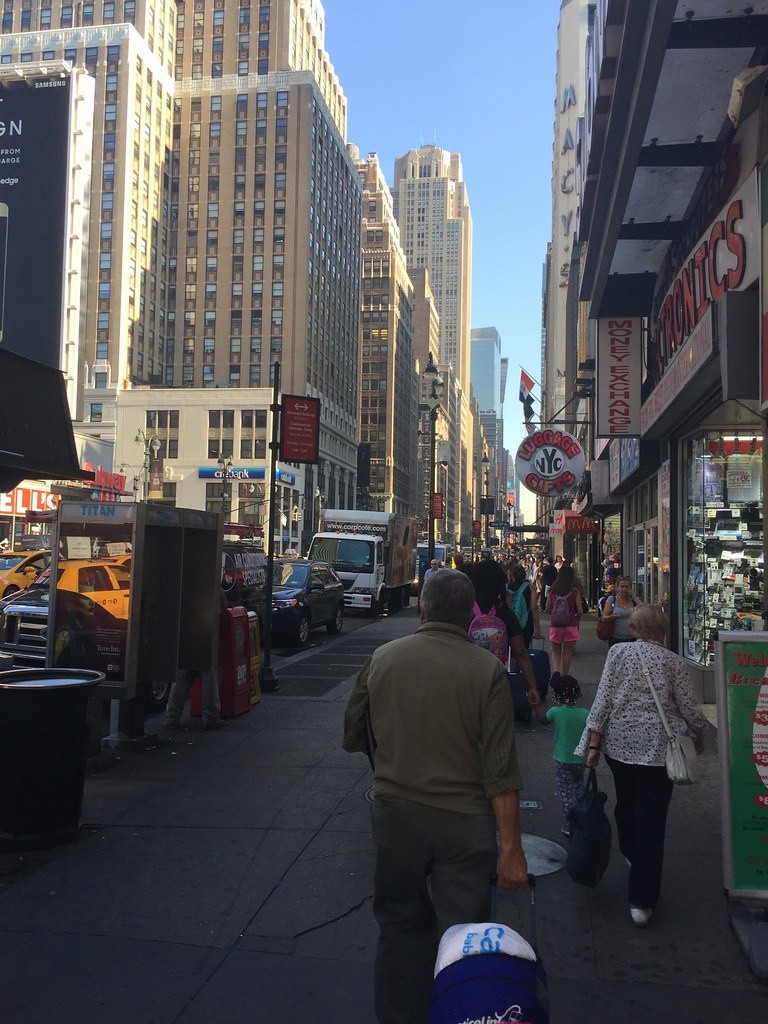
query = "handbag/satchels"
[666,732,700,786]
[597,596,616,641]
[567,767,612,888]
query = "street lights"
[217,454,234,522]
[315,487,325,534]
[423,352,445,561]
[481,452,494,549]
[121,463,147,502]
[135,428,161,504]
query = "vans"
[409,541,452,597]
[223,539,267,609]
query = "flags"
[519,369,535,404]
[523,404,535,422]
[525,421,536,434]
[519,389,535,407]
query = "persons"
[467,560,539,707]
[419,548,579,613]
[574,602,708,930]
[528,674,596,837]
[545,565,583,691]
[602,575,643,651]
[340,569,531,1024]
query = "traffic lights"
[293,513,302,523]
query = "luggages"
[527,636,551,702]
[506,642,533,721]
[431,873,551,1024]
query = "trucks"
[307,509,418,617]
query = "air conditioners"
[187,376,193,381]
[206,349,210,353]
[188,349,194,354]
[254,348,261,352]
[276,320,281,325]
[277,348,281,352]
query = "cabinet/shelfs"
[688,499,767,663]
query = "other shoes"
[630,905,652,926]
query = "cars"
[57,559,129,620]
[0,549,51,599]
[99,555,132,568]
[270,559,345,647]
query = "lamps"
[700,433,764,459]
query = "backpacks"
[550,588,577,627]
[467,600,509,664]
[505,582,530,630]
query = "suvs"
[0,561,51,673]
